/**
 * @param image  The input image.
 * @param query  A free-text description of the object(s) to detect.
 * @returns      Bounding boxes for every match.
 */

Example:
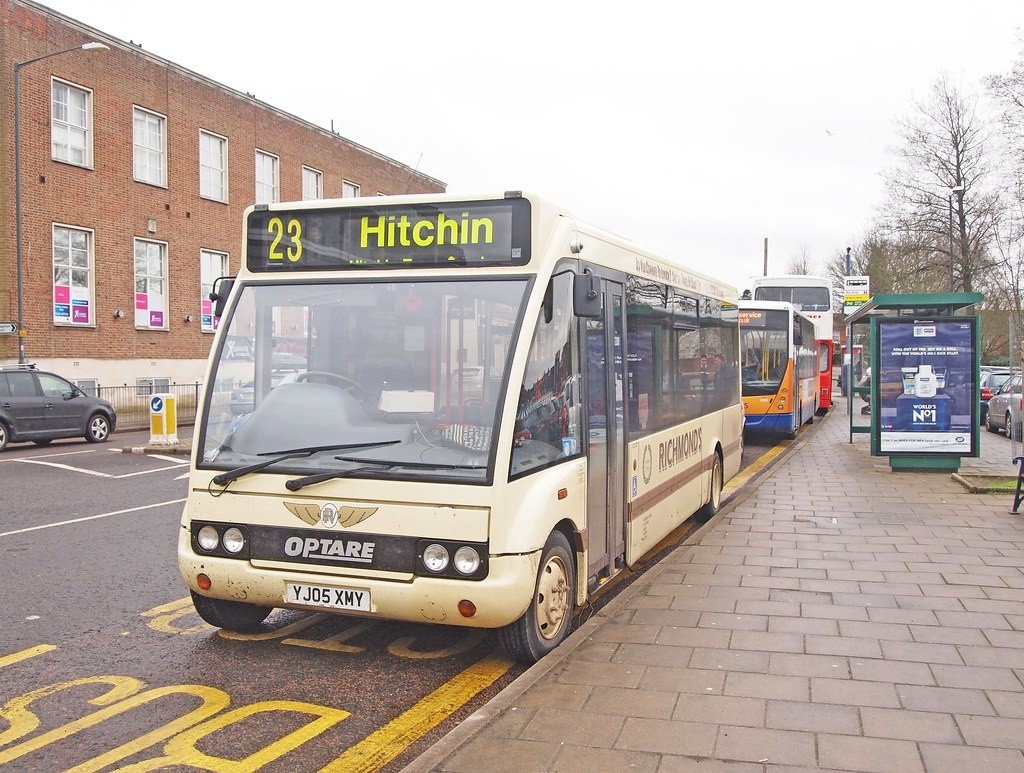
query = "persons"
[713,354,735,409]
[701,354,709,397]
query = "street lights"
[11,41,113,369]
[948,185,966,292]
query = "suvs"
[0,363,118,452]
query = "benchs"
[1009,456,1024,514]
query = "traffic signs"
[0,321,17,335]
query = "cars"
[980,365,1022,426]
[229,378,256,416]
[881,367,970,417]
[859,366,871,402]
[451,366,501,397]
[985,370,1024,439]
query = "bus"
[176,182,749,673]
[751,276,836,416]
[732,300,821,441]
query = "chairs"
[741,348,787,380]
